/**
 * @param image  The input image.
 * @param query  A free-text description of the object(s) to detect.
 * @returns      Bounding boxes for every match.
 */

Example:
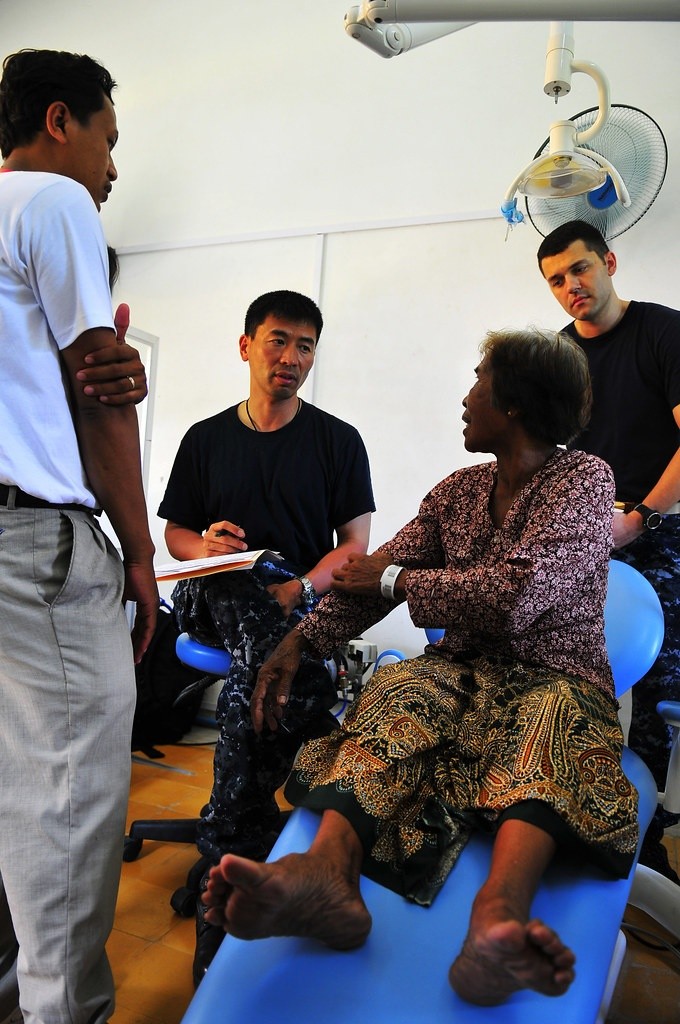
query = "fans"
[524,104,669,244]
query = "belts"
[0,484,92,513]
[613,503,680,514]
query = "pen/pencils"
[214,527,248,537]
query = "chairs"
[179,558,665,1024]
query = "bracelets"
[380,564,406,599]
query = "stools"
[120,633,236,919]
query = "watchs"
[298,575,316,606]
[633,502,663,530]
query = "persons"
[129,290,377,985]
[0,49,163,1024]
[199,332,639,1004]
[538,220,680,884]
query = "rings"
[127,376,136,390]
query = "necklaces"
[246,396,300,431]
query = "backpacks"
[129,600,205,751]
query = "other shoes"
[194,897,227,977]
